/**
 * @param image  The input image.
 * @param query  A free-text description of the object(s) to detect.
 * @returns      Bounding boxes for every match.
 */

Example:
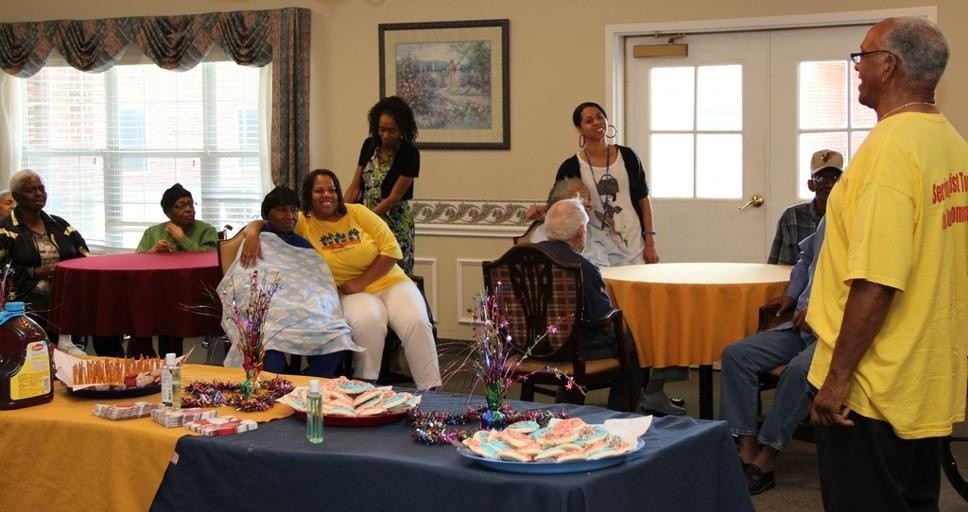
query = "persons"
[552,103,686,418]
[4,170,126,358]
[529,178,627,276]
[0,191,13,218]
[342,97,420,273]
[768,147,843,262]
[241,169,443,391]
[804,16,963,511]
[524,197,646,414]
[127,183,218,357]
[217,187,366,376]
[721,218,827,494]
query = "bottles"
[161,354,182,410]
[295,376,411,416]
[306,379,322,443]
[469,418,628,460]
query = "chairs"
[382,274,436,375]
[757,305,814,443]
[512,220,544,245]
[482,244,631,411]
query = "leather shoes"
[737,455,752,472]
[745,464,781,495]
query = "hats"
[810,149,844,176]
[160,183,192,214]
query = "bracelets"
[643,232,655,234]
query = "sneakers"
[637,387,687,418]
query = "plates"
[65,385,162,398]
[292,401,413,428]
[454,423,645,474]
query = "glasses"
[19,186,44,194]
[850,49,902,64]
[171,202,194,211]
[812,174,839,184]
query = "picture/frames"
[378,18,510,149]
